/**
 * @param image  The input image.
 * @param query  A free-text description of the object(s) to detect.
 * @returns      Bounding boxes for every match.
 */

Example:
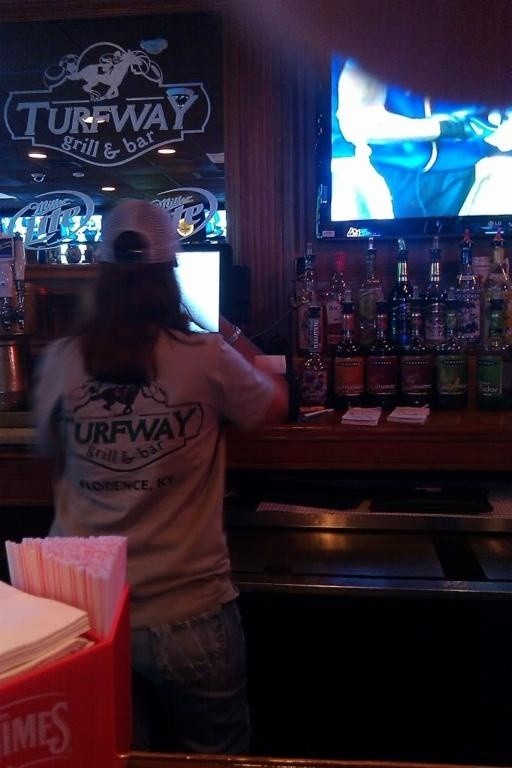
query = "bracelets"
[227,324,243,344]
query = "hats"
[95,198,176,266]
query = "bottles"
[291,231,512,412]
[65,240,81,263]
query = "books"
[387,403,431,427]
[341,405,383,426]
[298,404,334,416]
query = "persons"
[335,55,512,220]
[32,196,292,759]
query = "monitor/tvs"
[172,242,254,334]
[312,48,512,240]
